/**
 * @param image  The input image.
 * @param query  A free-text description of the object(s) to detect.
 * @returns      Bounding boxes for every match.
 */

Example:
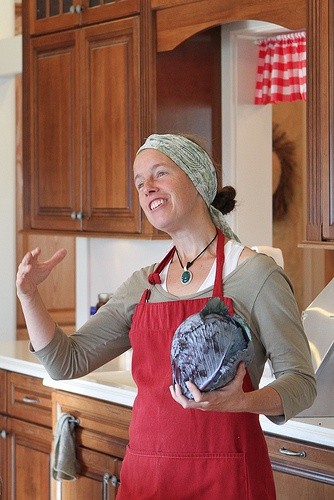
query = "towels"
[50,413,79,482]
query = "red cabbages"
[171,297,254,398]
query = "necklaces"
[173,230,219,285]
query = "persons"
[15,133,317,500]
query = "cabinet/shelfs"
[22,0,222,240]
[0,367,334,500]
